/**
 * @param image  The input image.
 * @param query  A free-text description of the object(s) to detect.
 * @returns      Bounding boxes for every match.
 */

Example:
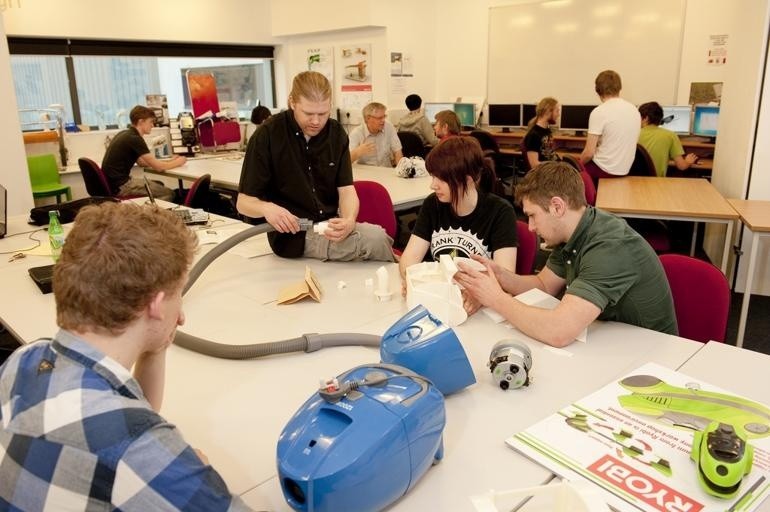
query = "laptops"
[143,176,208,225]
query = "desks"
[479,129,718,169]
[2,197,700,511]
[723,191,769,347]
[158,150,443,225]
[597,173,740,274]
[517,344,770,511]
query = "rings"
[341,235,344,239]
[471,301,475,306]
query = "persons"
[251,105,271,129]
[236,71,399,262]
[397,94,441,147]
[348,102,403,168]
[454,161,679,348]
[101,105,186,202]
[1,203,257,512]
[579,70,641,178]
[523,97,560,168]
[433,110,462,144]
[399,136,518,317]
[639,101,699,177]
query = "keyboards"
[498,143,519,148]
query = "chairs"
[179,172,214,208]
[77,157,110,198]
[516,222,541,282]
[29,153,75,209]
[656,246,730,342]
[356,180,400,246]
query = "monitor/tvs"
[454,103,476,128]
[559,105,598,137]
[424,103,455,125]
[489,104,520,133]
[659,105,692,135]
[693,106,720,144]
[523,105,537,126]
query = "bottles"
[46,210,66,264]
